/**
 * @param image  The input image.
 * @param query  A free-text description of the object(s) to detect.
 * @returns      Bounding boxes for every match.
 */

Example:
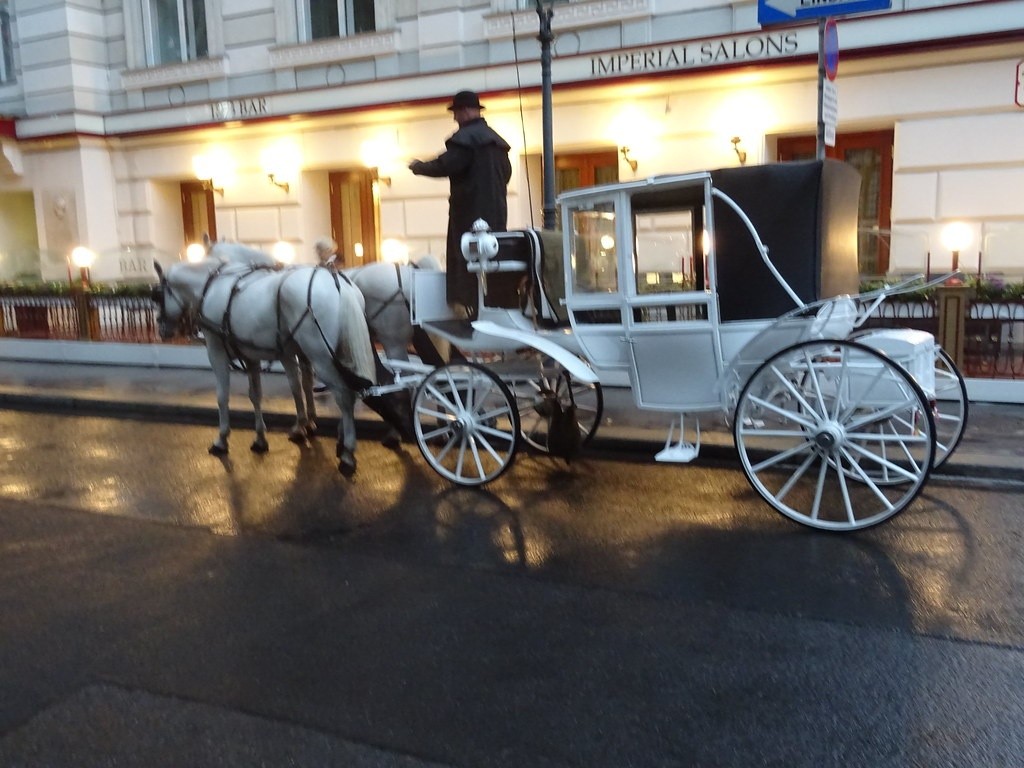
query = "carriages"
[154,157,971,531]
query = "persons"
[409,91,513,324]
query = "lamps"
[605,112,645,170]
[65,246,95,293]
[715,103,752,164]
[355,138,393,186]
[258,141,292,192]
[191,145,227,197]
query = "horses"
[153,234,442,477]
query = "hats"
[447,92,484,111]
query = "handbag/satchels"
[547,370,582,456]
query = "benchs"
[421,231,594,357]
[573,309,643,324]
